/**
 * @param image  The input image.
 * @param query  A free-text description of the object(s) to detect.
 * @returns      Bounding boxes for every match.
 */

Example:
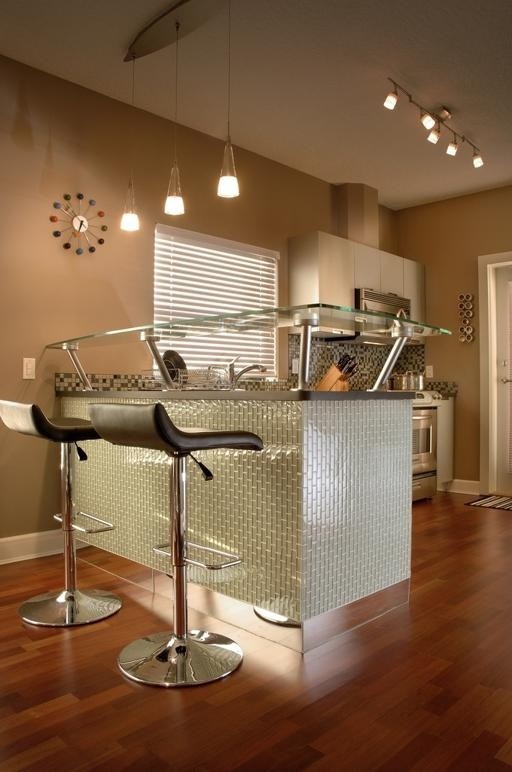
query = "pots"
[392,369,426,390]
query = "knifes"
[337,353,358,381]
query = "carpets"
[466,494,511,511]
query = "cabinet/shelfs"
[403,256,425,346]
[353,241,403,297]
[288,231,354,339]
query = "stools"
[86,403,263,691]
[0,397,123,626]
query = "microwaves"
[357,289,414,340]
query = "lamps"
[381,75,482,170]
[117,1,242,232]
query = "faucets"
[227,357,267,392]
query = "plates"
[161,349,189,387]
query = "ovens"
[411,407,440,476]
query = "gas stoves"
[383,390,433,404]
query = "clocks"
[47,190,109,254]
